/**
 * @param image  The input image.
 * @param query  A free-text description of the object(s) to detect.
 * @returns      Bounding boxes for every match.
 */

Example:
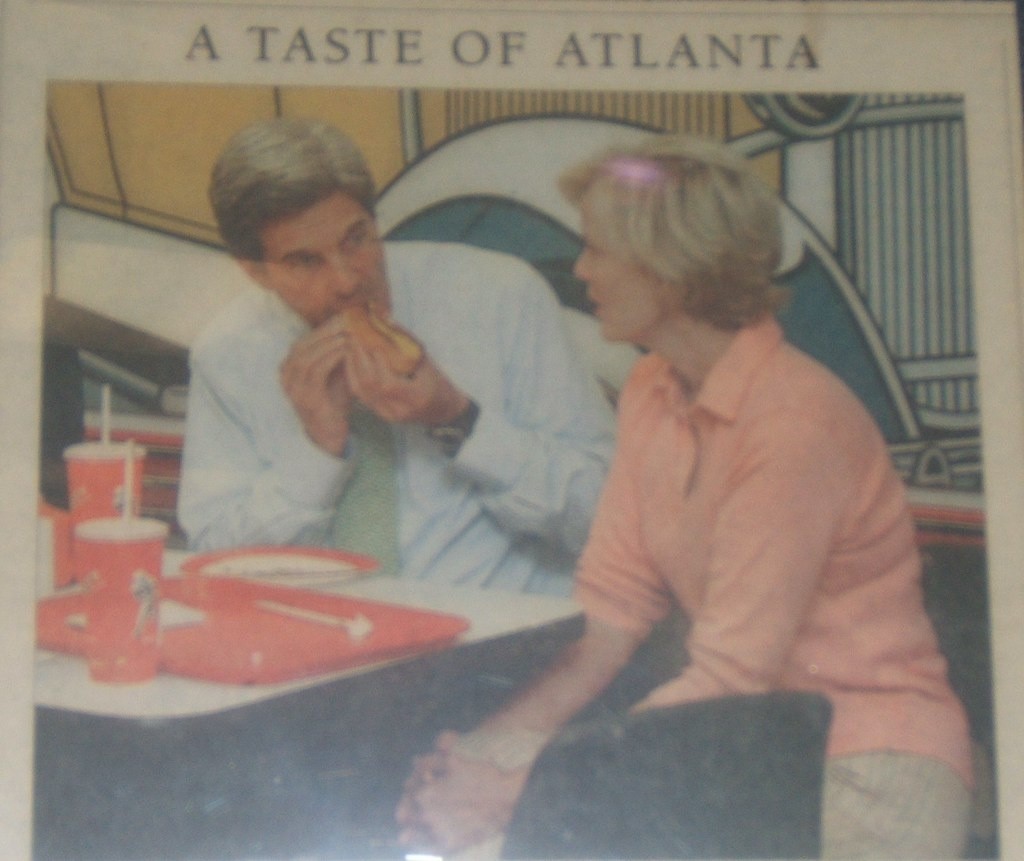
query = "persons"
[175,119,617,605]
[395,132,974,861]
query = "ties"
[330,400,401,575]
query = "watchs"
[428,400,478,458]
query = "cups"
[74,516,169,683]
[64,439,148,581]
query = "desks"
[31,514,586,860]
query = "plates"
[178,545,380,585]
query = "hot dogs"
[341,298,425,377]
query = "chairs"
[502,693,835,860]
